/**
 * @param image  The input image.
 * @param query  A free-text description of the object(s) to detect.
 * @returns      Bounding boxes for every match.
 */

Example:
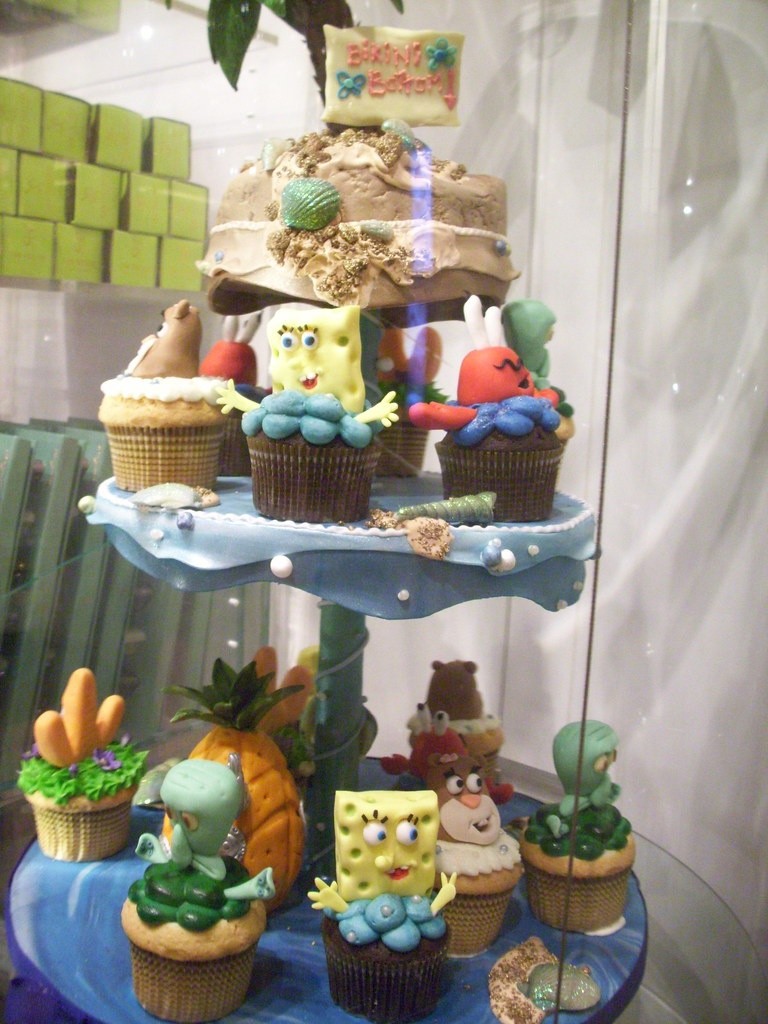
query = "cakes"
[199,118,520,311]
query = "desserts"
[98,295,574,523]
[15,640,638,1023]
